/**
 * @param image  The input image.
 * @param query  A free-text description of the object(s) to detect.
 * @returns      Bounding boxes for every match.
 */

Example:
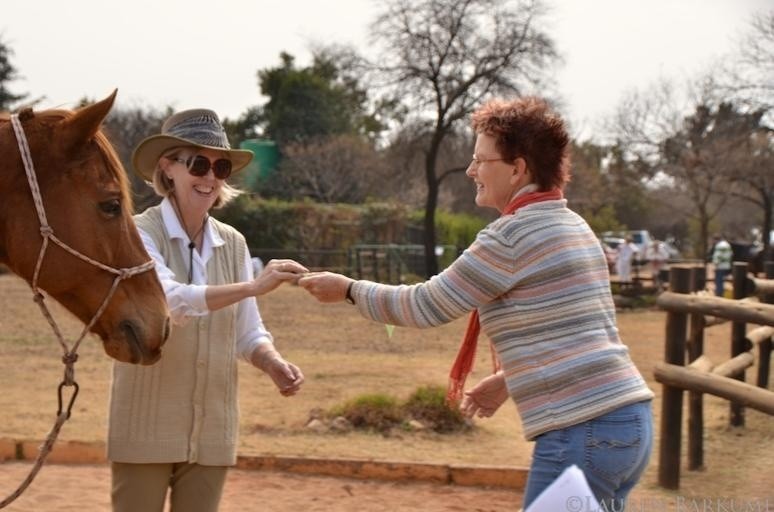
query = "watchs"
[345,281,354,306]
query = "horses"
[0,85,172,369]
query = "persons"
[712,233,733,296]
[297,96,655,512]
[109,108,305,512]
[642,241,668,289]
[615,235,638,288]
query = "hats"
[132,110,254,182]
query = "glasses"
[472,154,527,175]
[174,156,232,179]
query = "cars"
[598,228,682,271]
